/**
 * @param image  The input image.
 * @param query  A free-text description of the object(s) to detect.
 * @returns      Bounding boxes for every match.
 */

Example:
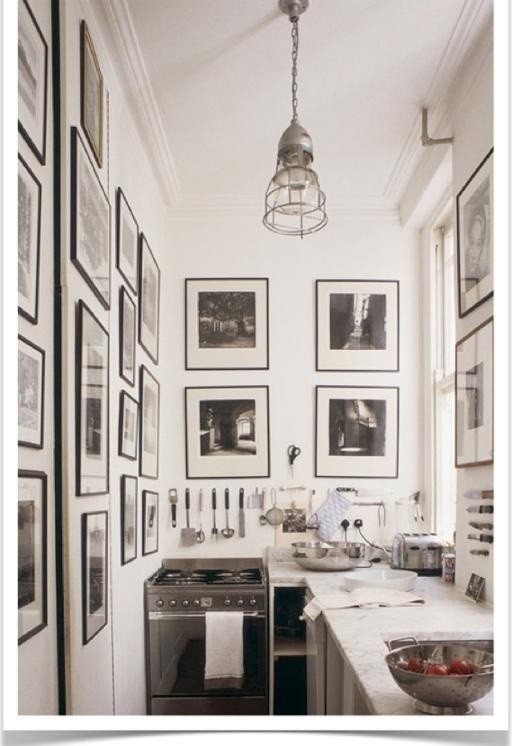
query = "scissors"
[287,445,301,478]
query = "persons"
[466,208,488,290]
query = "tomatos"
[408,659,474,676]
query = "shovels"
[182,489,195,546]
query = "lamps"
[263,0,328,239]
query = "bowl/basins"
[291,540,367,572]
[383,629,493,656]
[342,568,418,593]
[384,634,493,715]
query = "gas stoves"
[143,567,266,612]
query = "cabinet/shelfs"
[268,579,305,716]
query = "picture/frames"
[315,279,399,372]
[121,473,139,565]
[118,388,140,462]
[185,278,269,371]
[18,0,48,167]
[185,385,271,479]
[18,332,46,450]
[138,231,159,366]
[18,469,48,648]
[138,363,159,479]
[82,509,108,645]
[455,316,494,468]
[18,151,42,326]
[115,185,140,295]
[141,489,158,558]
[118,284,136,388]
[315,385,400,478]
[80,19,106,169]
[456,147,494,319]
[75,298,110,497]
[69,125,113,311]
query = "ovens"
[146,612,269,714]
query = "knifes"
[466,503,494,514]
[461,533,493,543]
[461,489,494,499]
[469,550,491,556]
[468,521,494,532]
[238,488,246,537]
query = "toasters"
[391,531,443,577]
